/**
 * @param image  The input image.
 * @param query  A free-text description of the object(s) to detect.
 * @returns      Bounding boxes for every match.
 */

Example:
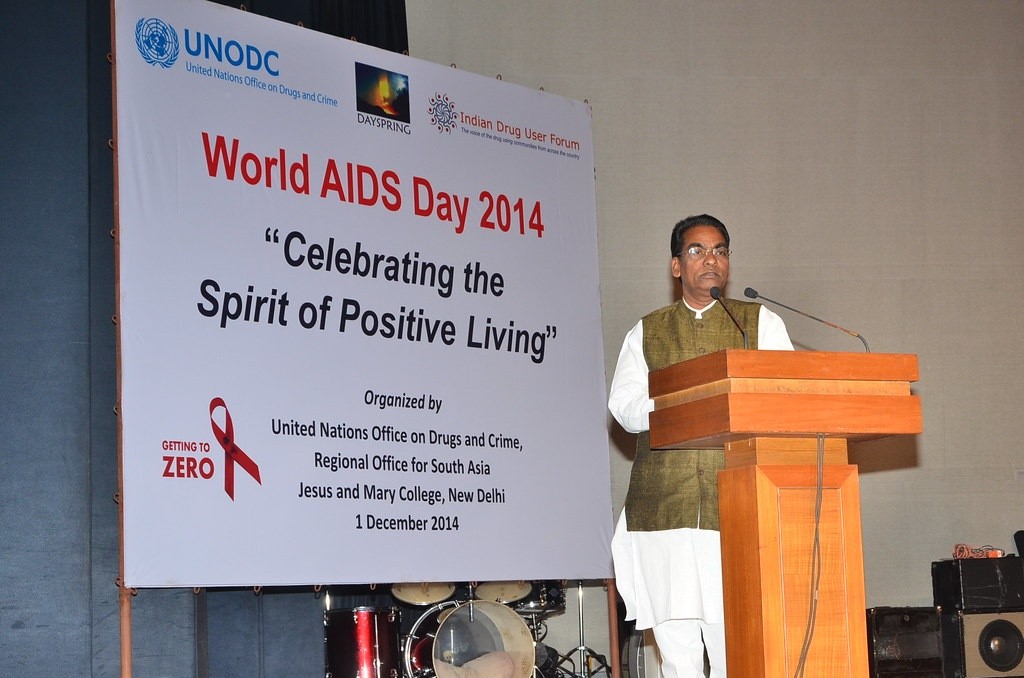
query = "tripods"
[528,580,612,678]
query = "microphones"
[744,287,870,354]
[710,286,748,350]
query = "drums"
[324,579,568,678]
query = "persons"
[607,214,796,678]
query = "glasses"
[677,247,732,258]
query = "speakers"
[866,553,1024,678]
